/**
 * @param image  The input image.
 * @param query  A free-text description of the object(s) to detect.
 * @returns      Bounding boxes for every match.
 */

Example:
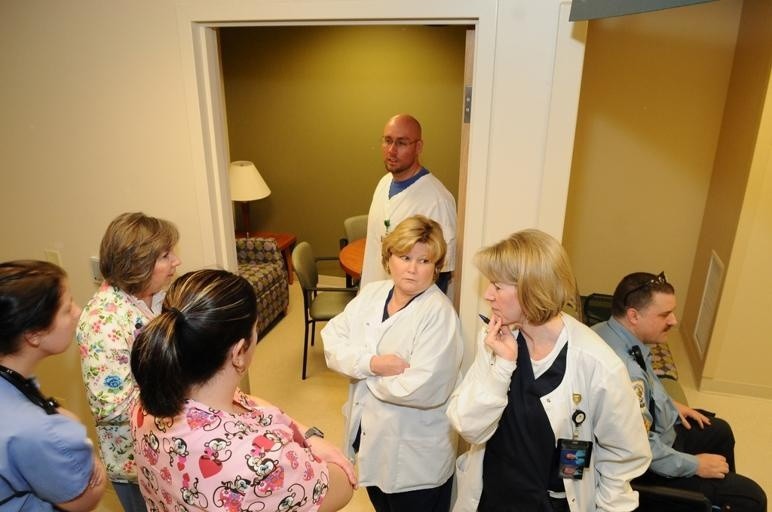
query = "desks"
[339,238,366,280]
[235,231,296,285]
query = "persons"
[563,466,582,479]
[76,212,181,512]
[565,450,585,465]
[0,259,108,512]
[320,215,464,512]
[129,269,359,512]
[357,113,457,306]
[445,228,652,512]
[588,272,767,512]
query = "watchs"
[305,426,324,439]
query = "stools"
[650,341,679,378]
[658,378,689,406]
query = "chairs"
[631,484,721,512]
[236,238,288,336]
[291,242,358,380]
[340,215,368,288]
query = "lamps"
[229,160,271,238]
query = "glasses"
[624,271,666,304]
[382,136,419,148]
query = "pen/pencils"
[479,314,503,336]
